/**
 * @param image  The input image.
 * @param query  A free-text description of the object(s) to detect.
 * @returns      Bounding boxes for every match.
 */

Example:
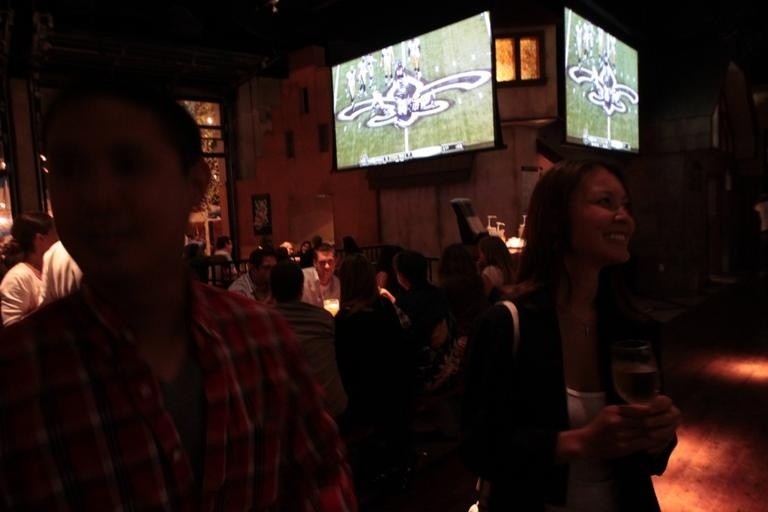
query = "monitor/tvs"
[329,8,509,174]
[555,0,643,161]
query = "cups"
[323,299,339,316]
[610,339,661,405]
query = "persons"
[0,212,56,326]
[183,235,516,449]
[575,20,620,73]
[340,36,421,121]
[36,242,84,308]
[0,67,359,512]
[458,158,682,512]
